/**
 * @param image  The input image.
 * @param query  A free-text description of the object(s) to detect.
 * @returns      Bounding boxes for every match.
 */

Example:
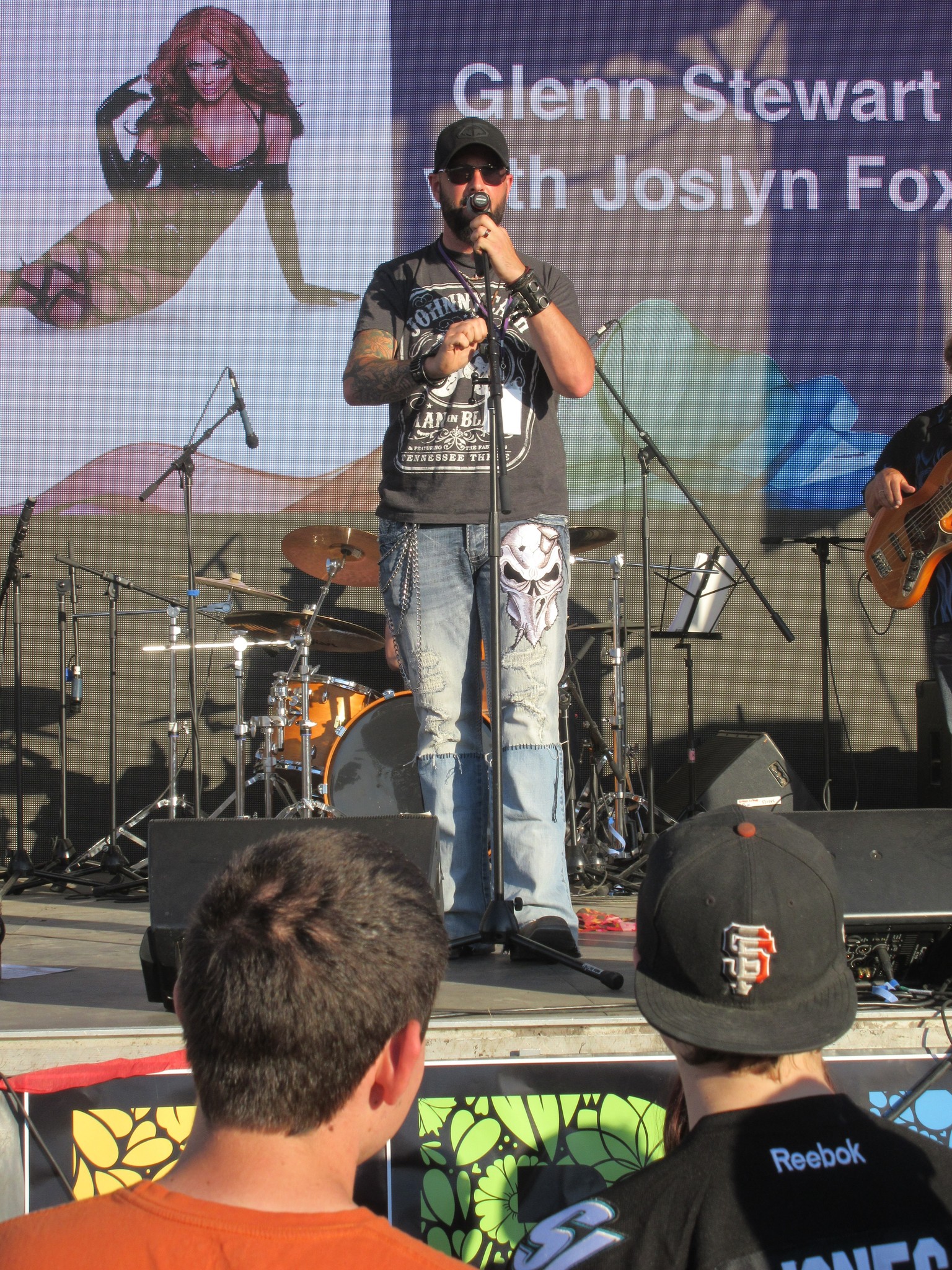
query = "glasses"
[438,164,510,186]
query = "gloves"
[262,184,361,307]
[93,75,158,202]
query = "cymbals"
[568,526,618,556]
[281,522,380,591]
[223,605,386,654]
[170,569,294,604]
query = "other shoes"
[446,915,576,962]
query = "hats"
[634,803,858,1055]
[434,116,511,174]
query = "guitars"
[862,447,952,611]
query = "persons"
[501,801,952,1270]
[339,111,603,966]
[0,6,363,331]
[860,330,952,704]
[0,821,491,1270]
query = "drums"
[255,668,382,776]
[322,688,492,858]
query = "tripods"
[649,546,753,823]
[0,456,348,902]
[438,271,630,993]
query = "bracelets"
[408,351,451,389]
[503,265,535,294]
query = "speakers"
[139,813,445,1012]
[663,730,819,813]
[771,808,952,1002]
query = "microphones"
[464,192,490,222]
[6,496,38,564]
[227,369,260,450]
[71,664,83,715]
[587,318,613,349]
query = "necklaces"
[447,252,506,320]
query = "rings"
[483,229,492,238]
